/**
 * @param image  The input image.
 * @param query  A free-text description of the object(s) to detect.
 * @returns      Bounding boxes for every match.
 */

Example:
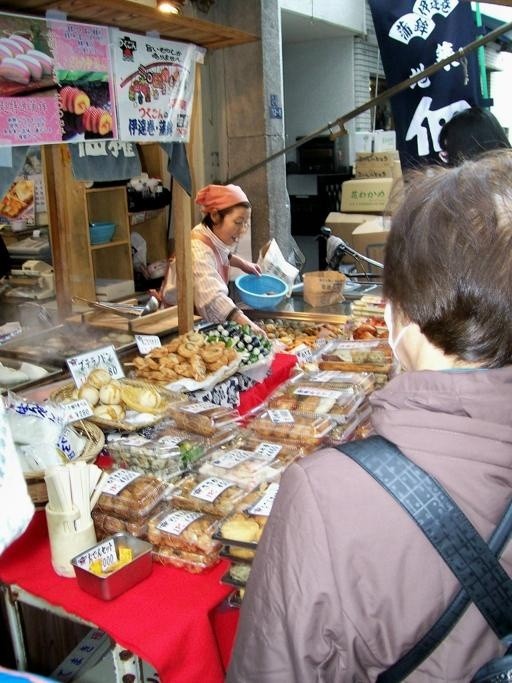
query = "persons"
[222,146,510,682]
[158,183,271,342]
[436,103,511,168]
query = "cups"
[45,503,99,579]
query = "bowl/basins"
[234,274,288,309]
[88,222,116,245]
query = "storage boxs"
[323,148,407,282]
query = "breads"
[72,315,398,601]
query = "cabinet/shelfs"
[69,184,169,306]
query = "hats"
[194,182,251,213]
